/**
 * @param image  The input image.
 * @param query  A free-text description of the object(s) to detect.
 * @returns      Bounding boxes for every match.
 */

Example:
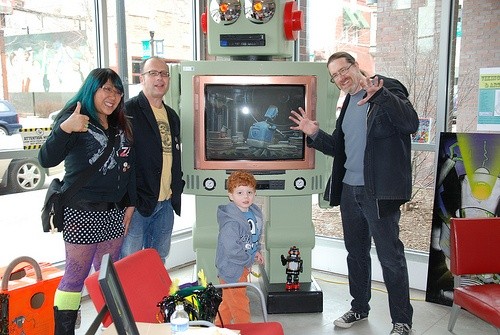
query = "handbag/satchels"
[41,178,63,233]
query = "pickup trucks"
[0,132,65,195]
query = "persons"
[37,69,137,335]
[119,56,186,265]
[214,170,265,326]
[289,51,420,335]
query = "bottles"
[170,302,189,335]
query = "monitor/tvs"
[193,74,317,170]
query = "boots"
[53,305,81,335]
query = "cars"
[0,99,23,136]
[48,109,62,124]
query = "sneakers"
[333,309,368,328]
[389,322,413,335]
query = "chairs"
[85,248,284,335]
[448,217,500,331]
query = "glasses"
[142,70,169,77]
[330,64,353,83]
[102,85,124,97]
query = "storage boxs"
[0,259,66,335]
